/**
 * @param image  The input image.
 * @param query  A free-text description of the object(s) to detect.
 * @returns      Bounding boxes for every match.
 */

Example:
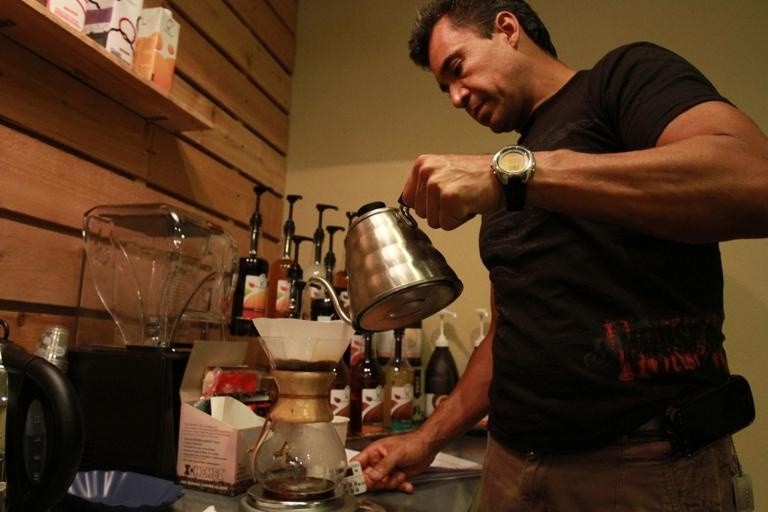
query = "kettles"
[0,314,84,510]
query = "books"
[342,444,486,486]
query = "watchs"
[488,142,536,215]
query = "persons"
[343,1,768,511]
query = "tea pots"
[306,200,464,335]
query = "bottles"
[251,369,347,496]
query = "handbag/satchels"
[487,370,689,456]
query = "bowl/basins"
[67,470,184,509]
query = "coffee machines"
[73,203,238,480]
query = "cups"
[34,323,71,371]
